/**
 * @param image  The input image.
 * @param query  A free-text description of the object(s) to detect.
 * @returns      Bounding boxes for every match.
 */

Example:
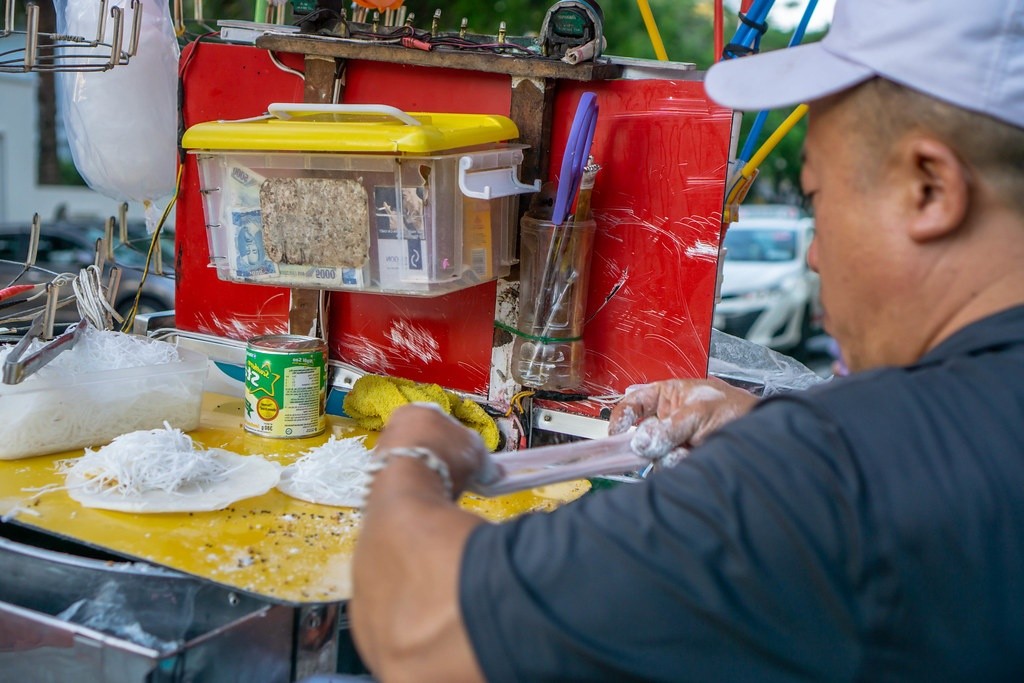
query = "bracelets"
[373,444,454,497]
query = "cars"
[712,202,825,367]
[0,221,177,333]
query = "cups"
[511,210,596,388]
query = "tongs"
[1,268,122,387]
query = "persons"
[349,1,1024,683]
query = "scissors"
[527,93,599,348]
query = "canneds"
[243,335,329,439]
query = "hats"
[704,1,1024,125]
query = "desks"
[0,392,591,683]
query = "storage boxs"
[181,100,542,298]
